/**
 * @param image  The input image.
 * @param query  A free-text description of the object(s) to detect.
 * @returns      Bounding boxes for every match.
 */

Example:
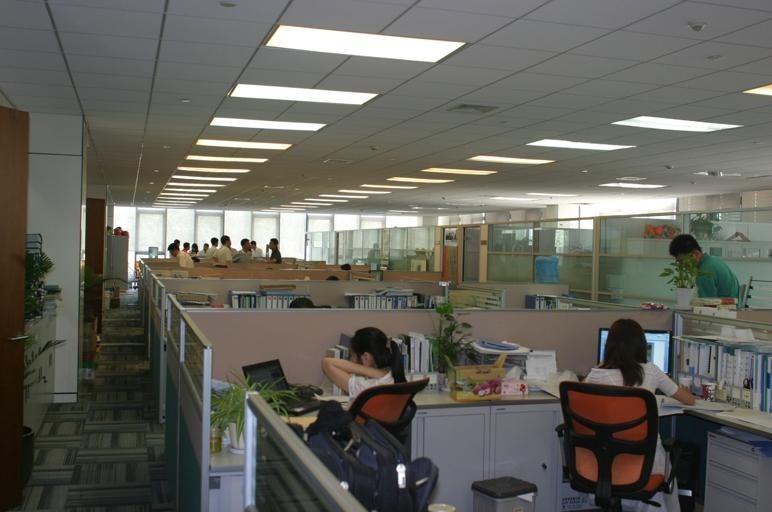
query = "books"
[678,340,771,412]
[325,330,433,396]
[230,289,256,309]
[406,292,435,308]
[690,298,741,319]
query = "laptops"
[241,359,322,416]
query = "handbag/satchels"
[309,419,439,511]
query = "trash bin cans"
[471,476,537,512]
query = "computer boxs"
[663,439,697,512]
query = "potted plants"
[210,371,299,455]
[659,254,714,311]
[22,252,64,485]
[426,302,474,392]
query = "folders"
[765,355,772,413]
[526,295,545,309]
[352,296,418,308]
[232,295,310,309]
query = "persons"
[168,235,281,266]
[322,327,408,409]
[582,318,695,512]
[669,234,739,298]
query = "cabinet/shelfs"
[626,237,772,259]
[412,391,562,512]
[703,427,772,512]
[107,235,128,289]
[23,313,56,433]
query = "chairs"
[352,376,429,424]
[553,380,665,512]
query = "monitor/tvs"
[597,328,672,381]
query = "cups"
[678,378,692,394]
[702,383,717,402]
